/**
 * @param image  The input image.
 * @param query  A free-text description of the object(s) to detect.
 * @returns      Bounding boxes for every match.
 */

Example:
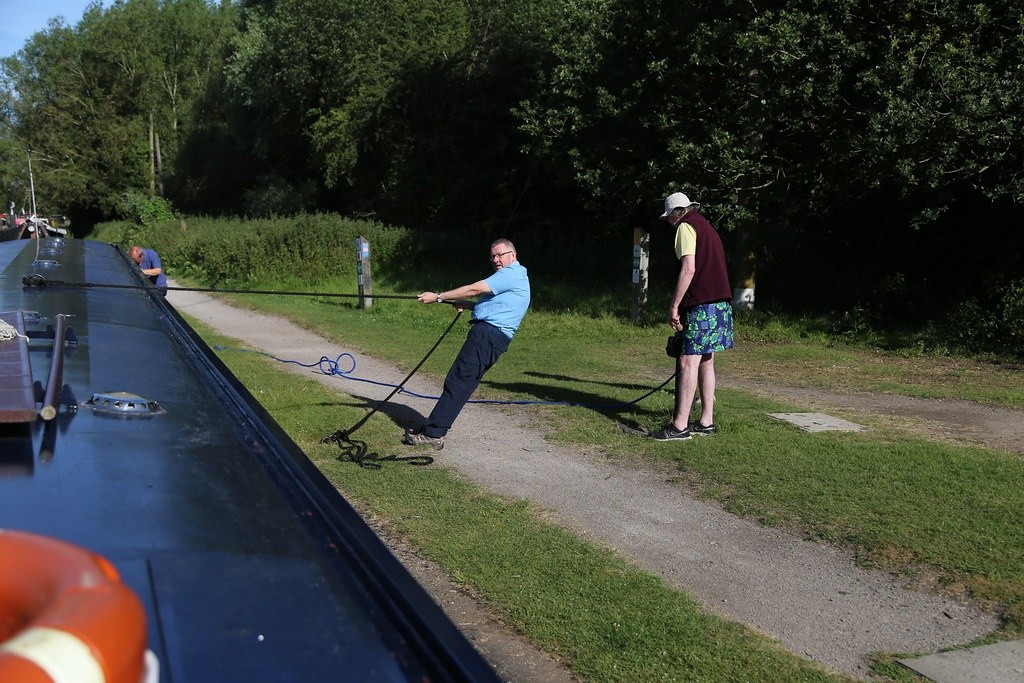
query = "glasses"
[489,251,513,261]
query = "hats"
[658,192,700,219]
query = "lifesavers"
[0,524,148,683]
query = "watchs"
[436,292,442,303]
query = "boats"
[0,234,504,683]
[0,151,68,243]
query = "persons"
[127,246,167,297]
[406,239,531,446]
[647,192,735,440]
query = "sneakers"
[408,427,447,439]
[406,432,444,450]
[648,422,692,441]
[687,420,716,436]
